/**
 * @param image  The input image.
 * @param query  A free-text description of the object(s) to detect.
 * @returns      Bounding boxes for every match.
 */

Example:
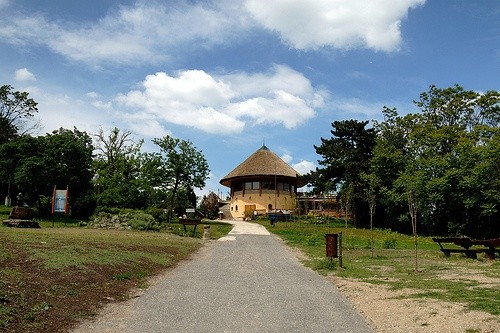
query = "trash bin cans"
[325,233,340,258]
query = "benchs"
[432,237,500,260]
